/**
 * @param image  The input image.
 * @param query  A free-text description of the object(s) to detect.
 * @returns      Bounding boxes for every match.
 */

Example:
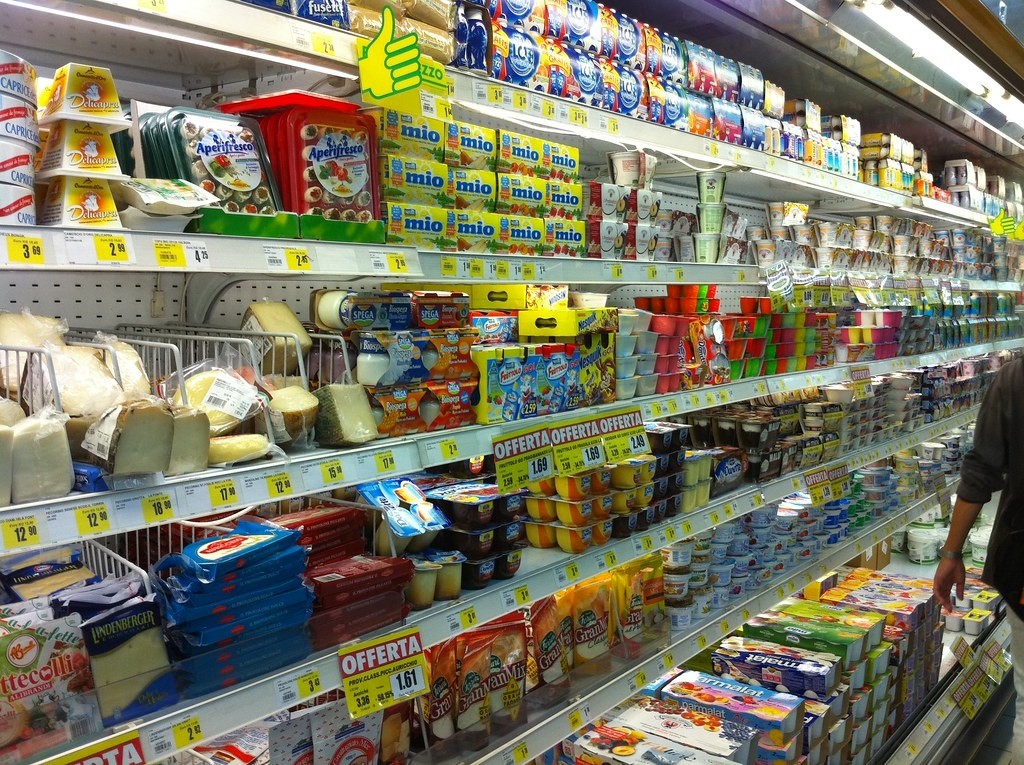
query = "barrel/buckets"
[969,526,994,567]
[890,505,951,565]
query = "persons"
[933,356,1024,765]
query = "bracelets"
[936,544,962,560]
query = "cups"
[682,448,712,514]
[744,198,1024,284]
[605,150,641,187]
[678,170,726,264]
[338,477,464,610]
[654,206,674,262]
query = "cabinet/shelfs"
[0,0,1023,765]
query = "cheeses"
[0,300,380,507]
